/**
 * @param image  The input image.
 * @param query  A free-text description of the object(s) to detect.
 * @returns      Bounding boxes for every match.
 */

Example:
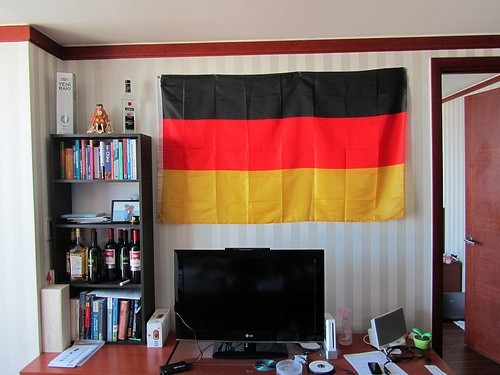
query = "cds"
[308,359,336,375]
[252,359,277,371]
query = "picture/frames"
[111,200,140,223]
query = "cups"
[276,359,303,375]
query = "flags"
[156,67,407,225]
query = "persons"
[92,104,110,132]
[121,202,138,220]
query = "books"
[70,287,143,343]
[60,210,112,224]
[59,137,138,180]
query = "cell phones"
[159,361,191,375]
[368,362,382,375]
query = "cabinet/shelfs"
[46,134,155,349]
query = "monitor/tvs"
[174,247,325,358]
[367,307,407,350]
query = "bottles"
[120,80,137,134]
[65,227,141,284]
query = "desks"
[20,331,457,375]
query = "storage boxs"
[56,72,77,134]
[147,308,171,349]
[41,284,71,352]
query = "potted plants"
[412,328,433,350]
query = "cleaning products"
[335,306,353,346]
